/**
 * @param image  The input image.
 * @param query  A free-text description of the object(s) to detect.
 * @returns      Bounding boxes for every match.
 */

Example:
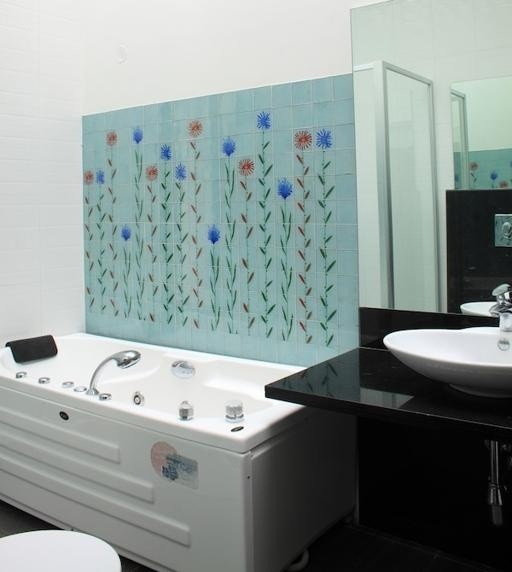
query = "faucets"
[496,291,512,331]
[489,283,511,316]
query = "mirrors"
[350,0,512,318]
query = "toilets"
[0,530,122,572]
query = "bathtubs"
[0,333,310,454]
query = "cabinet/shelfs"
[264,389,512,571]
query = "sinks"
[460,301,500,317]
[383,327,512,398]
